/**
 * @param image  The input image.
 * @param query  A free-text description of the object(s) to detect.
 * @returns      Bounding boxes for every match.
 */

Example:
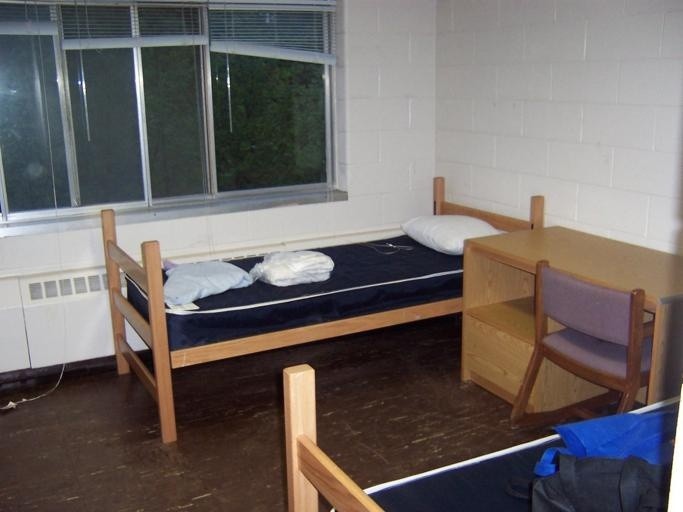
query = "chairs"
[508,257,653,429]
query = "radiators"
[19,243,286,371]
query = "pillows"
[400,214,508,257]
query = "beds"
[281,363,682,512]
[99,176,544,443]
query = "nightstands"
[460,225,683,408]
